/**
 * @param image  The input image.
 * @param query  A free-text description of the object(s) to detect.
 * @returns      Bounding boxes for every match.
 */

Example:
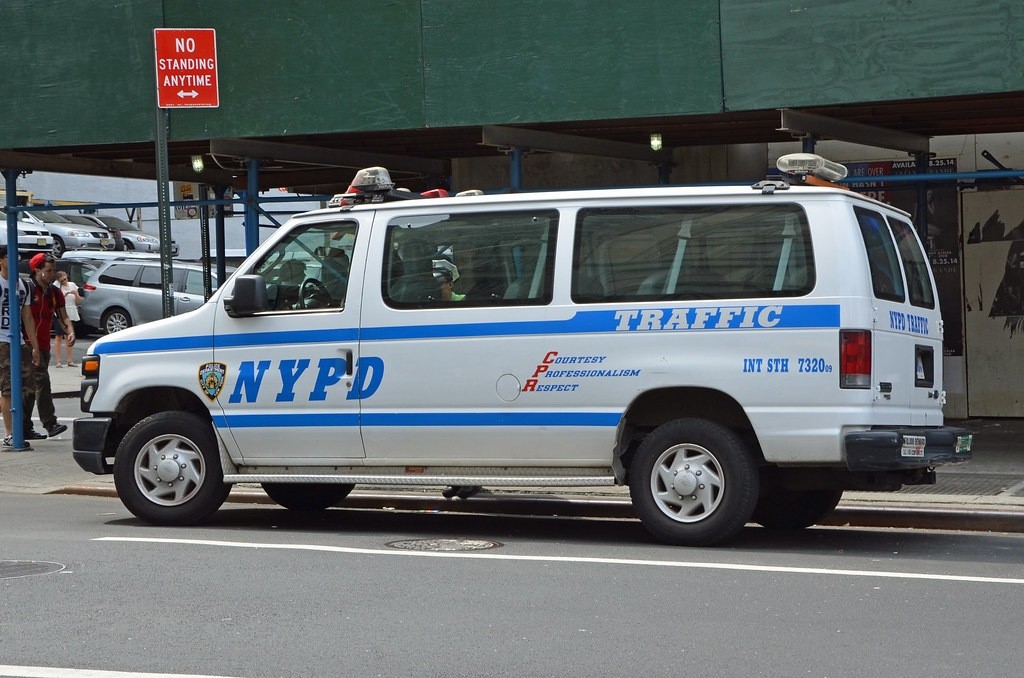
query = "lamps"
[190,151,205,174]
[650,132,663,151]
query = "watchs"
[288,303,294,309]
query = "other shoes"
[55,362,62,368]
[24,430,47,439]
[46,423,67,438]
[68,360,77,367]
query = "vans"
[71,152,980,548]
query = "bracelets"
[70,332,75,336]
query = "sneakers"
[2,434,30,448]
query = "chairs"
[502,243,545,300]
[790,266,808,291]
[720,270,773,294]
[636,258,685,296]
[451,237,506,301]
[577,274,605,295]
[391,239,441,301]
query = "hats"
[29,253,47,277]
[433,259,459,282]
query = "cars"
[86,212,180,258]
[28,249,326,336]
[0,207,55,260]
[29,210,117,260]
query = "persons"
[266,253,349,310]
[20,251,76,440]
[432,258,466,302]
[0,245,41,448]
[55,270,82,368]
